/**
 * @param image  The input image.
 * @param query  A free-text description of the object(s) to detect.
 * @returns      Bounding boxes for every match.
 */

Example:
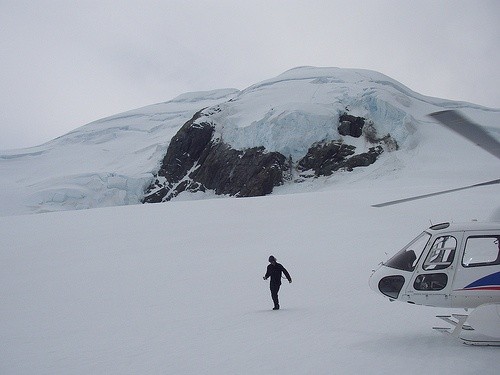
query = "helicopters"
[368,219,500,346]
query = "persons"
[263,255,292,310]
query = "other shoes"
[273,304,279,310]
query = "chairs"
[426,248,457,286]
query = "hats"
[268,255,277,262]
